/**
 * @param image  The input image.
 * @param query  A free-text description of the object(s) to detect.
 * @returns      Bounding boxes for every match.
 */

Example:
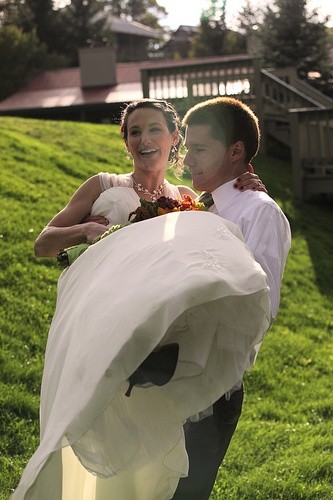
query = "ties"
[197,193,215,209]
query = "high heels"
[125,342,180,399]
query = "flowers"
[128,194,207,224]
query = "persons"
[175,97,293,500]
[31,98,203,402]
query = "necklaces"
[132,176,167,201]
